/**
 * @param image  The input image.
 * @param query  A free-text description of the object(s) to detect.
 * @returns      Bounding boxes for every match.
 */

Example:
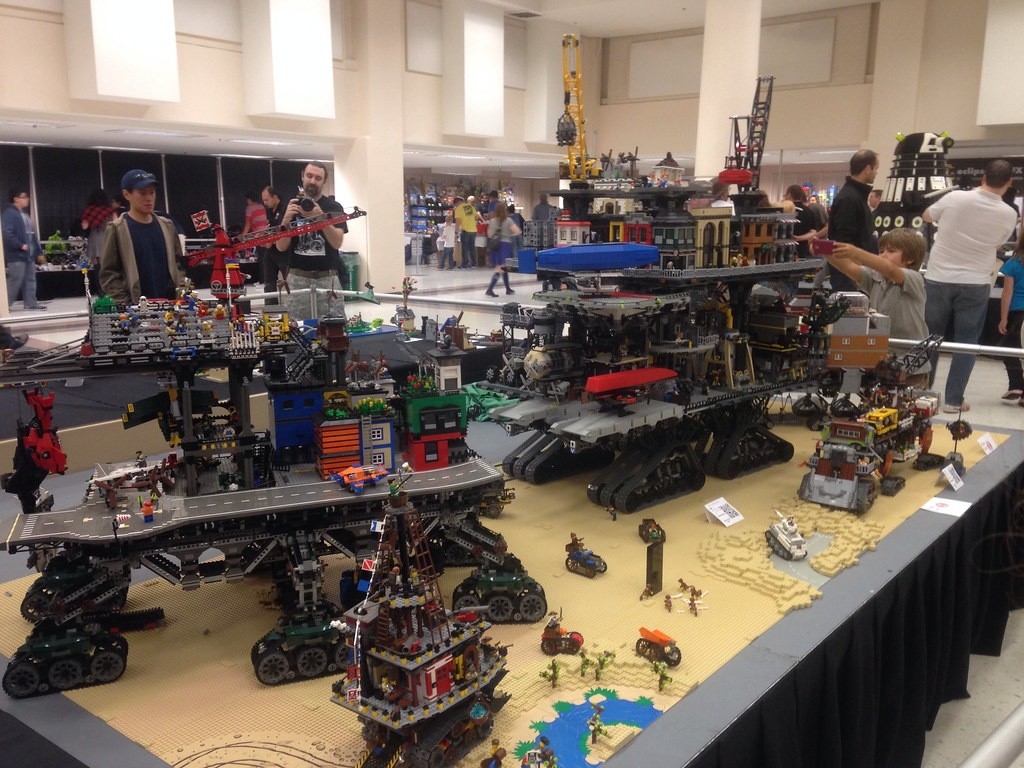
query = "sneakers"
[1018,398,1024,407]
[22,304,48,312]
[942,400,970,414]
[1002,389,1024,400]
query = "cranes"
[185,206,367,318]
[719,75,777,191]
[557,35,604,180]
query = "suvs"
[638,519,666,543]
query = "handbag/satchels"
[488,233,501,252]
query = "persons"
[262,186,286,305]
[487,191,499,215]
[453,197,477,270]
[570,532,578,545]
[476,192,489,224]
[532,195,552,220]
[467,195,477,209]
[507,205,525,248]
[922,160,1018,414]
[438,215,456,271]
[140,500,153,523]
[811,228,932,385]
[240,191,270,286]
[998,223,1024,407]
[782,150,883,292]
[115,282,227,339]
[223,424,235,435]
[0,188,46,310]
[490,739,500,756]
[486,203,522,297]
[81,188,118,296]
[275,161,348,320]
[112,196,129,217]
[99,170,185,303]
[710,176,736,216]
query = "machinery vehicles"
[541,624,584,656]
[765,509,807,561]
[565,543,607,578]
[251,600,357,685]
[21,550,132,622]
[452,556,547,625]
[2,616,129,698]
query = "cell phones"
[813,240,836,256]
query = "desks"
[35,269,96,302]
[187,263,259,289]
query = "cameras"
[295,196,315,212]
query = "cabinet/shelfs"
[409,204,451,234]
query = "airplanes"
[329,463,389,494]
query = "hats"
[120,169,161,190]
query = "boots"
[502,272,515,295]
[486,272,499,297]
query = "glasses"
[17,195,31,200]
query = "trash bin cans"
[338,251,358,301]
